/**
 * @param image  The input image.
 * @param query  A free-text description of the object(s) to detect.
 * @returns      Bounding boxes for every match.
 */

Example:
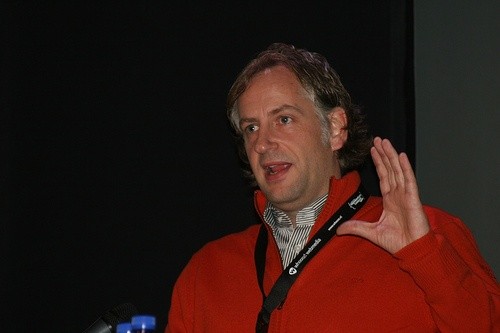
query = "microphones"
[88,302,136,333]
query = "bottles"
[116,323,132,333]
[131,316,156,333]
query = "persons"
[166,42,500,333]
